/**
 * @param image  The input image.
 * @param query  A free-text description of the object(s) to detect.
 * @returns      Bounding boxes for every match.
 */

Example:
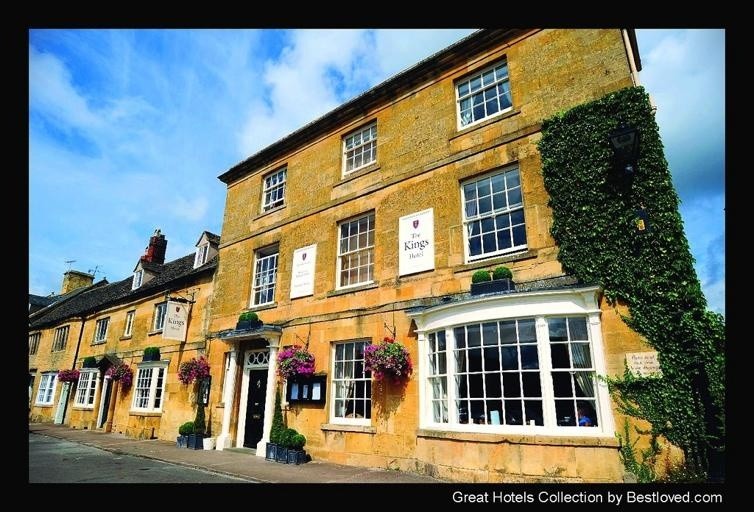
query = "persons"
[576,407,595,426]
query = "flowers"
[106,364,134,392]
[176,355,212,384]
[360,338,413,387]
[55,369,80,382]
[275,346,318,380]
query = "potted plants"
[236,311,263,329]
[142,347,161,361]
[469,267,516,296]
[176,382,217,451]
[82,357,97,368]
[264,384,306,465]
